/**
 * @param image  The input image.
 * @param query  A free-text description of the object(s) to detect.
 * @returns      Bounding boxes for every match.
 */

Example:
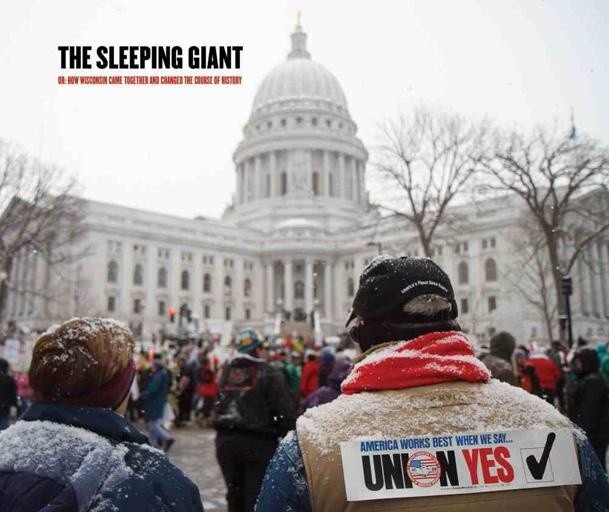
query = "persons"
[0,252,609,512]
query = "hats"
[234,328,269,354]
[344,255,459,328]
